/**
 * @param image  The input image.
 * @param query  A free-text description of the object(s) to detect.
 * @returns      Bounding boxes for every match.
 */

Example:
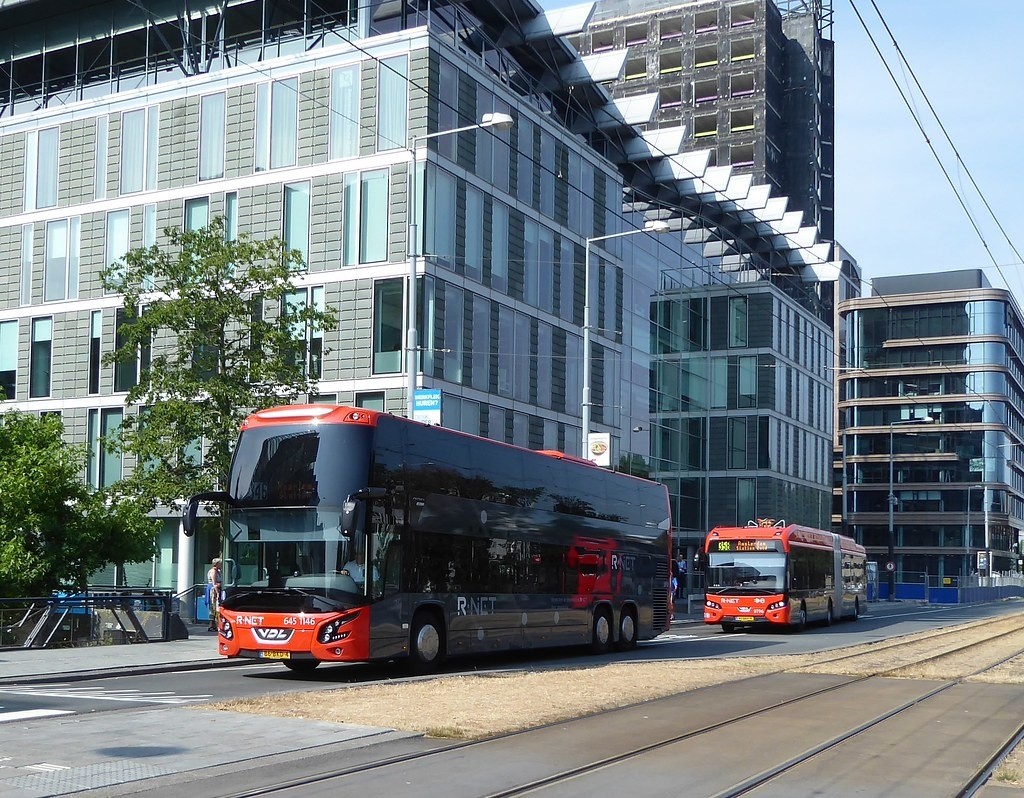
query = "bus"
[539,534,622,607]
[693,520,869,633]
[184,402,673,674]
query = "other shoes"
[680,597,686,599]
[670,614,675,620]
[208,627,218,632]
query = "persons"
[340,543,379,590]
[206,558,225,632]
[671,554,686,623]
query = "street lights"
[584,220,671,460]
[407,113,516,421]
[965,484,982,589]
[888,416,935,601]
[845,561,852,591]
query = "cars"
[842,583,864,594]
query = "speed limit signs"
[886,562,895,572]
